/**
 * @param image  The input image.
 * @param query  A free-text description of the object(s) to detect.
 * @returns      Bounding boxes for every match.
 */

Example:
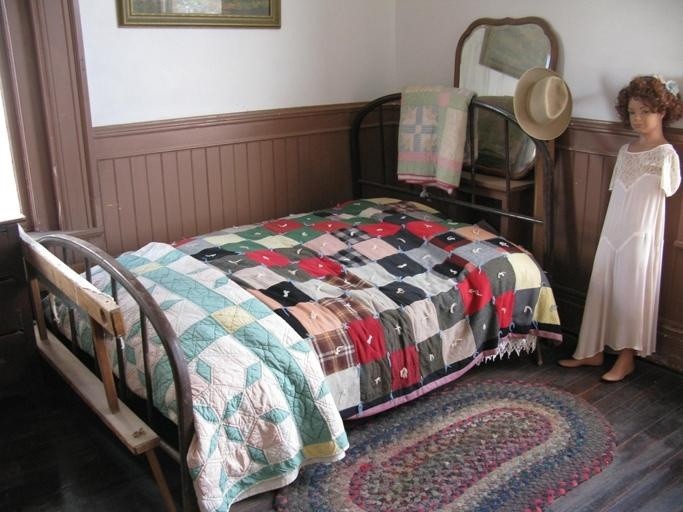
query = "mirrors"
[453,16,562,181]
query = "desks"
[446,166,535,246]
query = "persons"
[557,76,682,382]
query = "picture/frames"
[118,1,282,27]
[476,22,549,81]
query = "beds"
[18,87,551,511]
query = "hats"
[513,66,573,140]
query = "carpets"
[271,349,618,512]
